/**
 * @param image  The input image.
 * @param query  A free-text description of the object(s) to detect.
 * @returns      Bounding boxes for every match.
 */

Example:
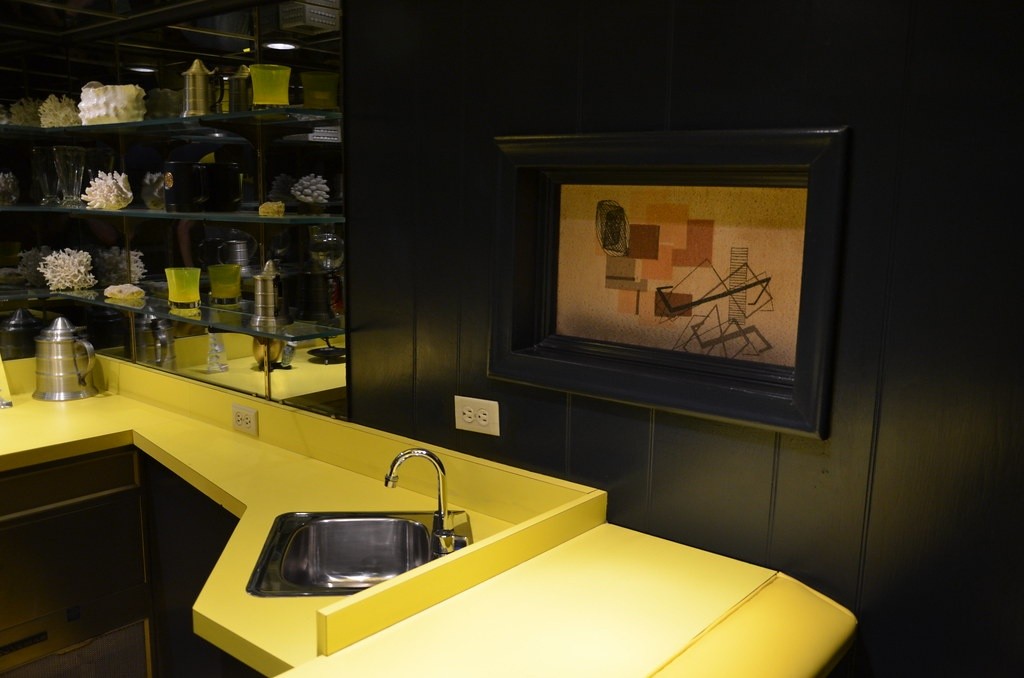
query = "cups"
[206,264,244,309]
[300,71,339,111]
[163,160,243,212]
[164,266,203,313]
[249,63,291,119]
[30,144,88,208]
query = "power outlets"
[232,404,259,437]
[455,396,499,436]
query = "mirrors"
[0,0,346,418]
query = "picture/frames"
[486,124,851,439]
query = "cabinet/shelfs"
[0,125,113,340]
[58,108,345,341]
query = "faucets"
[383,448,455,554]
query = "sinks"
[245,509,474,599]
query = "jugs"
[250,261,290,328]
[125,305,178,373]
[179,58,225,117]
[30,316,101,401]
